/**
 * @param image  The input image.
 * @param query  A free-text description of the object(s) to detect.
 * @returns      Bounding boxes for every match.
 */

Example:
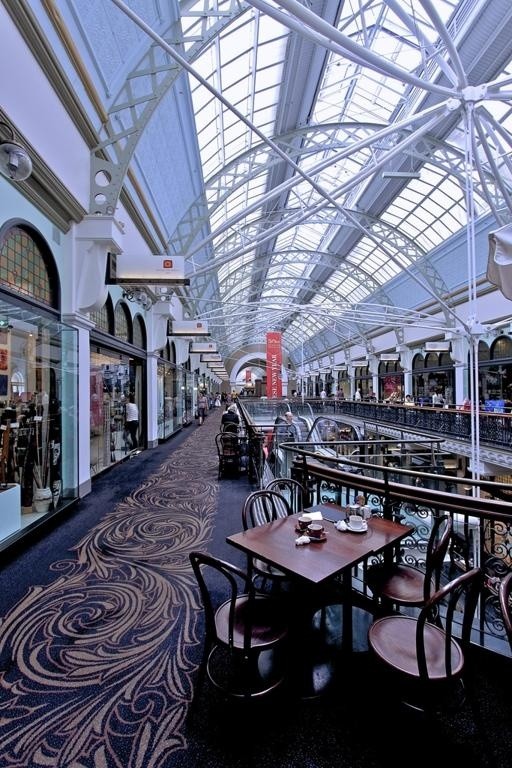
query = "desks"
[224,500,416,657]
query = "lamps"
[122,289,147,304]
[0,121,32,181]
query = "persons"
[222,408,239,424]
[279,411,298,442]
[431,390,443,406]
[403,395,414,404]
[337,388,344,397]
[210,390,237,407]
[320,389,326,398]
[198,392,208,426]
[355,388,361,400]
[127,395,140,451]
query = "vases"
[34,488,52,513]
[0,483,21,541]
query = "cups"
[298,516,312,530]
[349,516,366,529]
[306,524,324,537]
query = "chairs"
[263,478,307,523]
[498,570,511,643]
[366,511,457,639]
[242,488,302,599]
[211,413,250,484]
[364,563,487,758]
[186,549,291,723]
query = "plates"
[295,525,309,533]
[303,531,327,542]
[345,522,368,532]
